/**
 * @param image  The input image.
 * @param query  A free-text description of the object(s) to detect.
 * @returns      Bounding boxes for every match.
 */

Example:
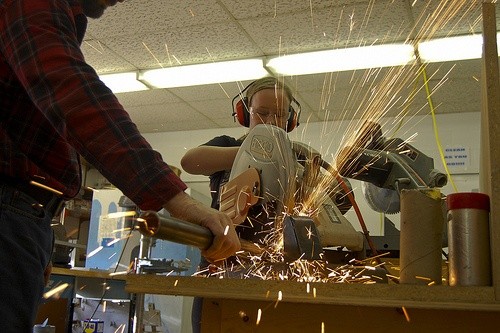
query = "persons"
[180,77,306,333]
[0,0,242,333]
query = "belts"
[0,177,67,219]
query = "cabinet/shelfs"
[55,186,93,266]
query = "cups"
[446,192,492,287]
[33,325,55,333]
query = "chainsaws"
[216,125,359,258]
[336,122,447,257]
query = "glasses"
[249,107,291,123]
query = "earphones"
[232,79,302,133]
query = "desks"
[122,271,500,332]
[48,265,135,333]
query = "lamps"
[100,32,484,93]
